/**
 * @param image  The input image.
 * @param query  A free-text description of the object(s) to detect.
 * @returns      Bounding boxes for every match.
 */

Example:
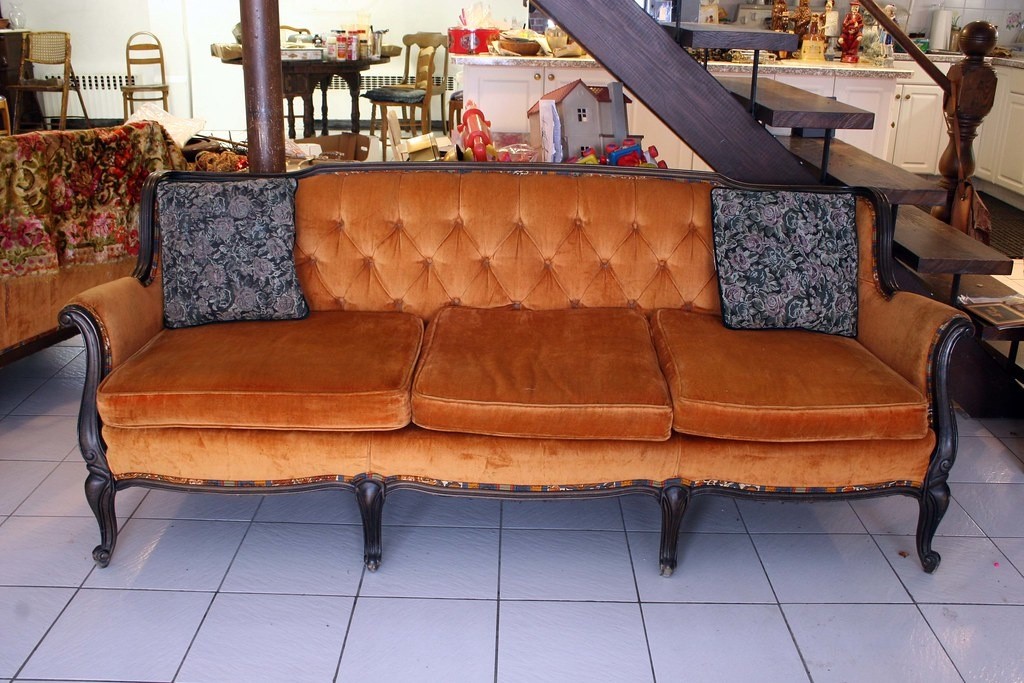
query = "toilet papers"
[929,9,953,50]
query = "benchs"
[57,161,976,577]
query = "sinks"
[925,49,963,56]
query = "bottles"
[312,30,369,63]
[20,75,63,85]
[907,31,928,54]
[9,1,26,29]
[802,14,826,62]
[773,13,796,60]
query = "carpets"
[915,191,1024,259]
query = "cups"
[447,26,499,55]
[728,49,776,64]
[653,0,672,21]
[371,32,382,58]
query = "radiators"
[39,72,145,130]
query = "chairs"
[7,31,93,135]
[360,31,464,160]
[121,30,170,124]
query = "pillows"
[711,186,860,338]
[157,178,310,330]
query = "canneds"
[336,30,359,61]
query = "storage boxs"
[281,49,324,62]
[894,38,930,54]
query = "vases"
[950,26,961,52]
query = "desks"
[222,58,390,138]
[0,29,35,130]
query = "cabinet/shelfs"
[887,54,1024,211]
[456,59,914,172]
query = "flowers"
[952,10,960,26]
[1005,10,1024,32]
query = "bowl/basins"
[500,28,542,55]
[0,18,8,29]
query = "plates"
[930,48,961,55]
